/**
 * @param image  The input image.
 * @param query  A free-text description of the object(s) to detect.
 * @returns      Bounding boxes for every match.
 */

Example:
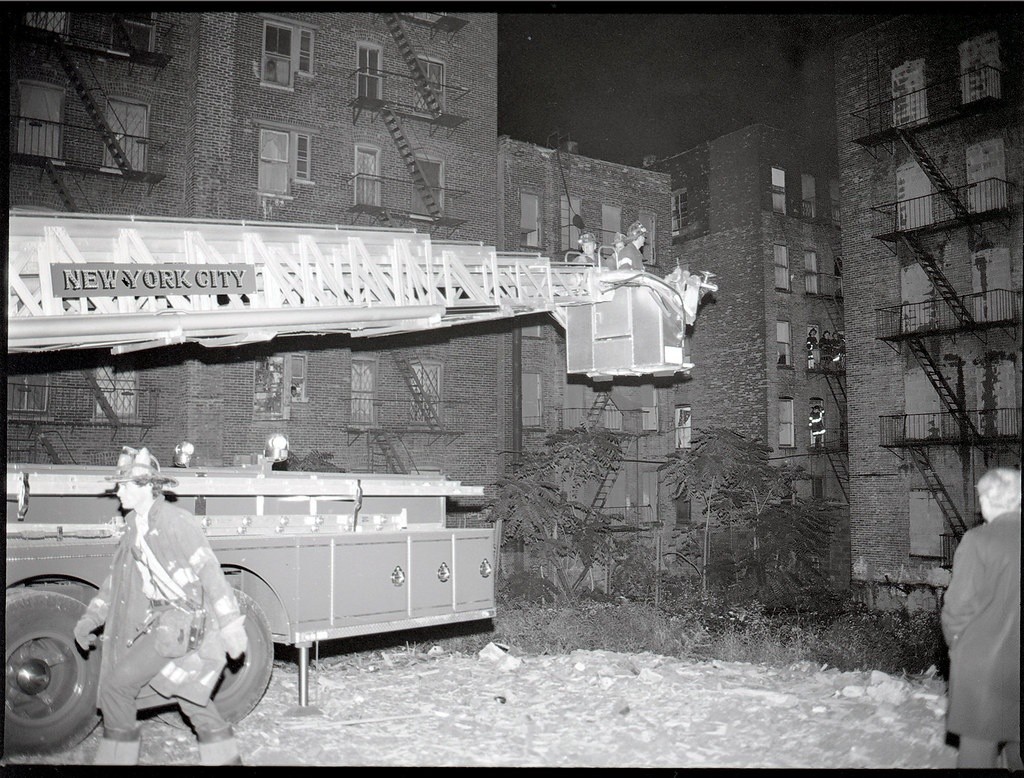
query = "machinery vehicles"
[4,204,717,755]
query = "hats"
[104,446,179,488]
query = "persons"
[809,406,825,450]
[618,220,647,271]
[820,331,845,370]
[806,329,819,368]
[74,446,248,765]
[942,469,1021,768]
[606,233,626,270]
[573,234,606,268]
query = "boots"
[194,723,243,764]
[92,729,142,765]
